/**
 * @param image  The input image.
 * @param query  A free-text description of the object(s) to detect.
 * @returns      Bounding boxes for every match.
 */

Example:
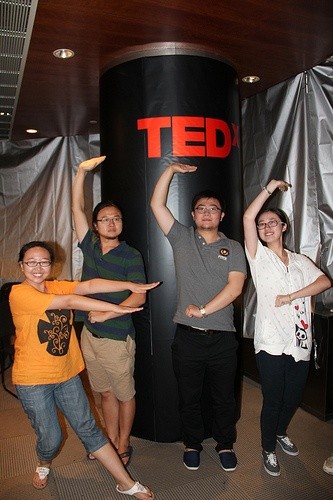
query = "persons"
[9,240,162,500]
[72,155,147,467]
[149,163,247,472]
[243,179,330,476]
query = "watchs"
[199,304,208,318]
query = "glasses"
[97,216,123,225]
[194,206,222,214]
[22,261,51,266]
[257,220,284,229]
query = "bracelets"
[263,185,272,196]
[288,293,293,304]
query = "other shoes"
[219,449,237,471]
[183,447,200,471]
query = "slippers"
[117,452,129,466]
[32,463,51,489]
[117,481,154,499]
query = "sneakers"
[261,450,280,476]
[277,435,299,456]
